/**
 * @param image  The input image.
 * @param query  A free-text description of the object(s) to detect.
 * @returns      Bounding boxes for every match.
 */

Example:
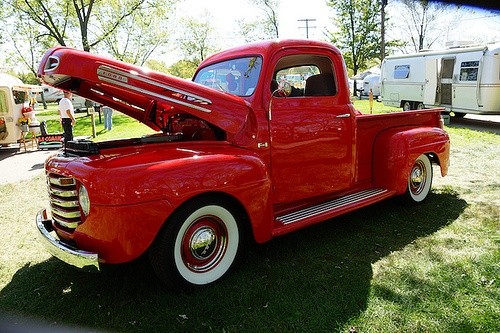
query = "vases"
[23,112,31,117]
[22,125,30,132]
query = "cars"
[26,82,106,108]
[363,72,381,97]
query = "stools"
[20,131,40,153]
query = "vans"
[0,72,29,145]
[378,45,500,118]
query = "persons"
[58,90,76,141]
[102,105,114,130]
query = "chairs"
[305,73,335,95]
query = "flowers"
[19,117,31,125]
[22,107,32,112]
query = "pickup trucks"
[36,39,450,288]
